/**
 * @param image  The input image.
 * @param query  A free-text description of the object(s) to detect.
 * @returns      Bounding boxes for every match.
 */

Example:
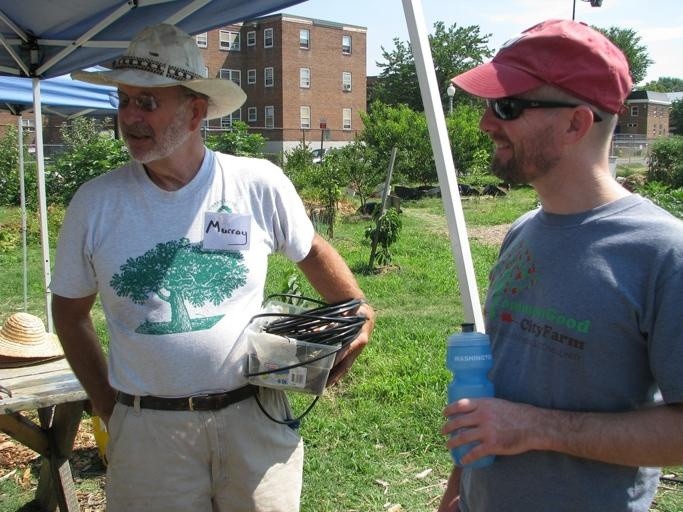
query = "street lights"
[571,0,604,27]
[447,82,456,114]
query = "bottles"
[442,320,498,470]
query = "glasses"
[489,96,603,122]
[109,90,196,111]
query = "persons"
[44,27,376,512]
[433,18,683,512]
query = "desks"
[1,359,89,511]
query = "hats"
[449,19,632,114]
[0,313,65,368]
[70,24,247,121]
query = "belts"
[117,384,264,411]
